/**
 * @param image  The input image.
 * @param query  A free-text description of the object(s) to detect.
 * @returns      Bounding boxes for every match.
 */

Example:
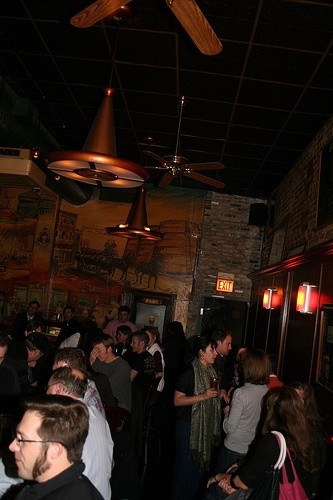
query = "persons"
[0,301,333,500]
[8,395,105,500]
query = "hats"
[26,333,50,351]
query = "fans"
[71,0,223,55]
[143,97,225,189]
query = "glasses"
[44,382,64,390]
[12,431,61,444]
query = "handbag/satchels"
[225,431,283,500]
[275,433,309,500]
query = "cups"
[210,378,219,398]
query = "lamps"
[295,282,318,314]
[48,15,144,190]
[262,288,279,309]
[106,136,164,237]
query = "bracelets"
[214,476,216,480]
[230,474,240,490]
